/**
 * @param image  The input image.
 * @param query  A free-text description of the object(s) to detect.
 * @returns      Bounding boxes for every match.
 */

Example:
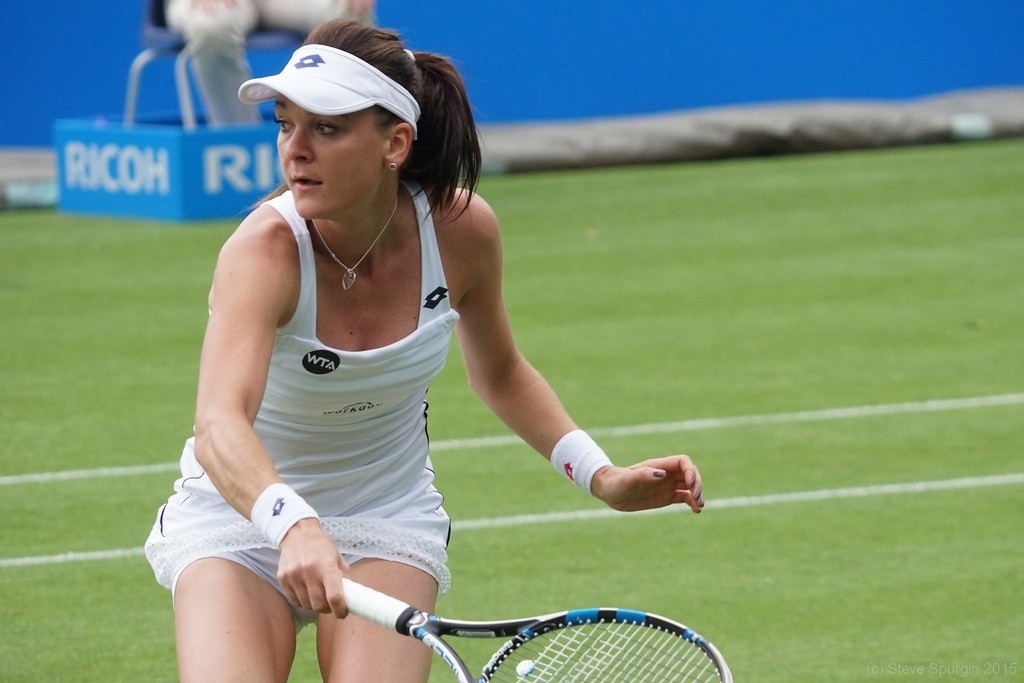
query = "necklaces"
[305,198,399,295]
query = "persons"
[143,21,705,683]
[146,0,377,126]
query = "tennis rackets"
[341,575,733,683]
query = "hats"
[237,44,421,143]
